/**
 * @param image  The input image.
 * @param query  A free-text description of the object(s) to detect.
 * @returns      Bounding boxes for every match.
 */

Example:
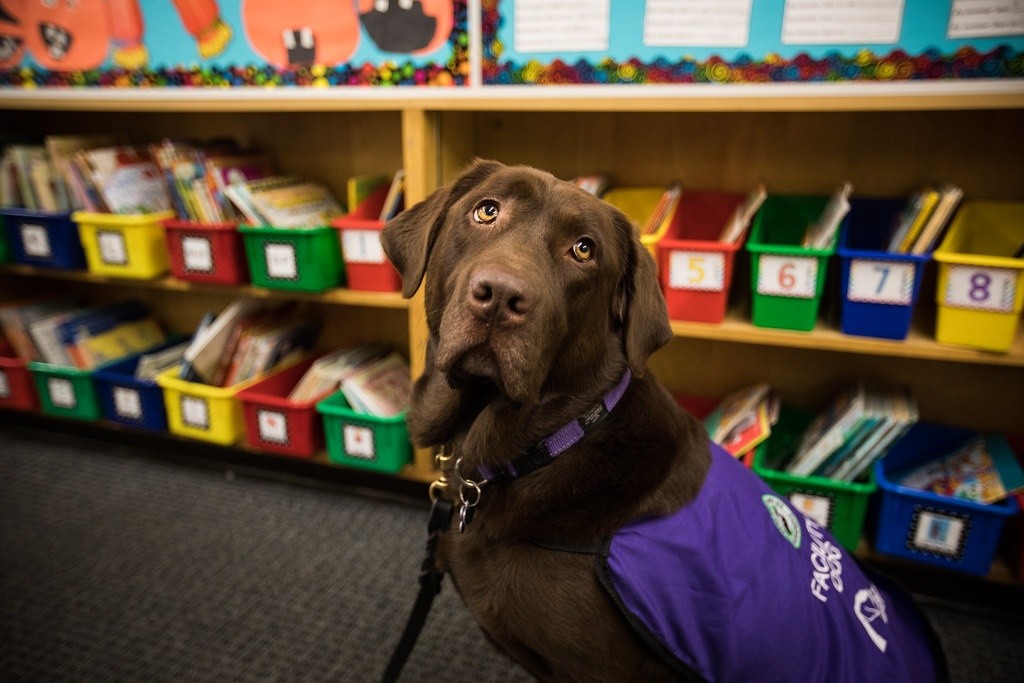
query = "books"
[0,134,410,421]
[573,173,1024,507]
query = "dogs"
[379,155,949,683]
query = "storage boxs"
[95,333,194,430]
[745,190,841,333]
[933,200,1024,355]
[154,345,318,445]
[333,181,402,293]
[28,329,184,420]
[0,206,86,271]
[658,191,750,324]
[598,185,681,278]
[876,420,1020,576]
[0,338,38,410]
[162,216,251,285]
[239,222,344,293]
[236,348,326,459]
[755,398,877,552]
[674,385,753,469]
[315,392,412,475]
[70,209,178,281]
[835,193,933,342]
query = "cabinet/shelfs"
[0,84,1024,592]
